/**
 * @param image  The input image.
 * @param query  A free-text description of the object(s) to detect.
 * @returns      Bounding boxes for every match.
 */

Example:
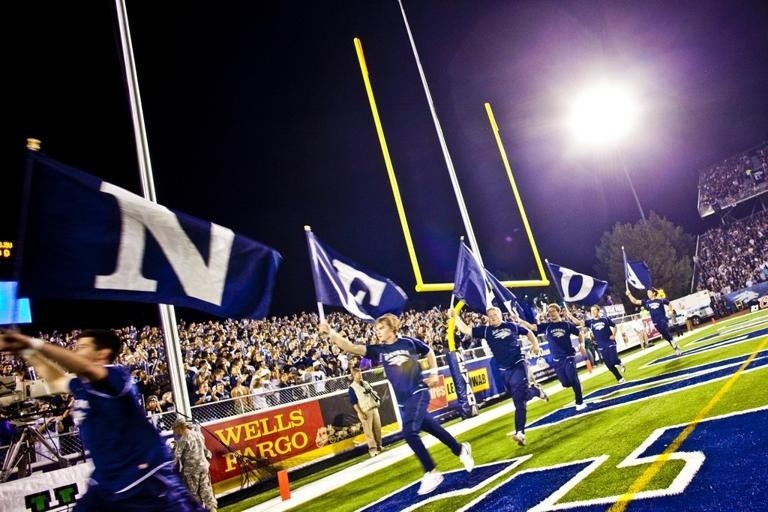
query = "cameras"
[363,389,371,394]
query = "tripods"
[6,439,59,476]
[1,422,61,482]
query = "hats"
[174,417,188,429]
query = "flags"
[452,240,536,325]
[621,249,652,290]
[306,231,408,324]
[545,262,608,305]
[6,150,284,322]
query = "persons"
[177,311,382,422]
[318,313,475,496]
[0,327,205,512]
[170,417,218,512]
[397,304,489,355]
[447,307,549,446]
[0,325,172,445]
[348,368,390,458]
[500,286,688,413]
[693,144,768,318]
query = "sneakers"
[417,346,680,496]
[369,447,389,457]
